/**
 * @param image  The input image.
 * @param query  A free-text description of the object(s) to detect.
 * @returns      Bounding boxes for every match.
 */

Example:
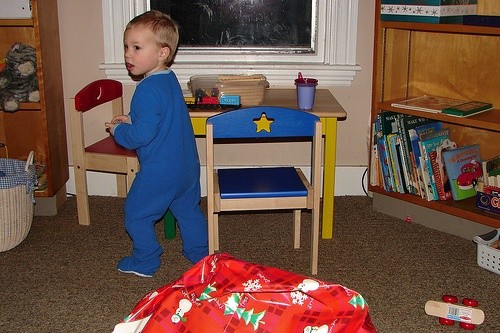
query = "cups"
[294,78,318,110]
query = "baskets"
[474,228,500,275]
[0,151,37,253]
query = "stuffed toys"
[0,42,40,111]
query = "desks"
[186,89,347,239]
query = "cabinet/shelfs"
[0,0,69,196]
[367,0,500,241]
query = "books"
[365,110,483,201]
[480,156,500,197]
[391,93,493,117]
[216,73,269,110]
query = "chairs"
[68,79,141,225]
[206,106,322,275]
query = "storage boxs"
[381,0,478,24]
[187,74,269,107]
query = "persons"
[104,10,209,278]
